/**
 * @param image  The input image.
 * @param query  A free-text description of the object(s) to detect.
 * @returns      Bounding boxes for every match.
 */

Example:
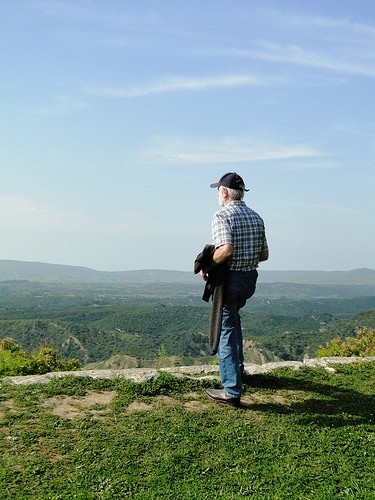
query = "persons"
[202,172,268,406]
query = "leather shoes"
[204,389,240,407]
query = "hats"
[210,172,250,192]
